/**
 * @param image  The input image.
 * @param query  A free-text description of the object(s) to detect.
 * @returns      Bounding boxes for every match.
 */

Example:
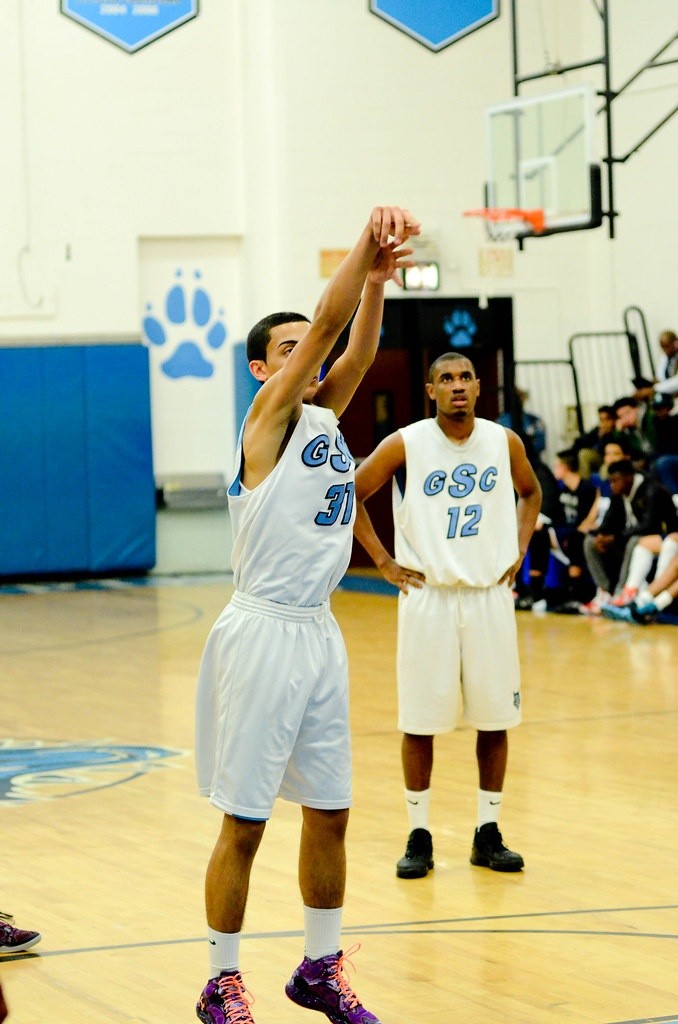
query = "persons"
[0,911,42,1024]
[494,331,678,625]
[197,202,421,1023]
[354,352,543,879]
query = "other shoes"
[578,588,661,625]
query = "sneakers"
[470,821,524,869]
[284,942,380,1024]
[196,971,258,1024]
[396,827,435,878]
[0,913,42,954]
